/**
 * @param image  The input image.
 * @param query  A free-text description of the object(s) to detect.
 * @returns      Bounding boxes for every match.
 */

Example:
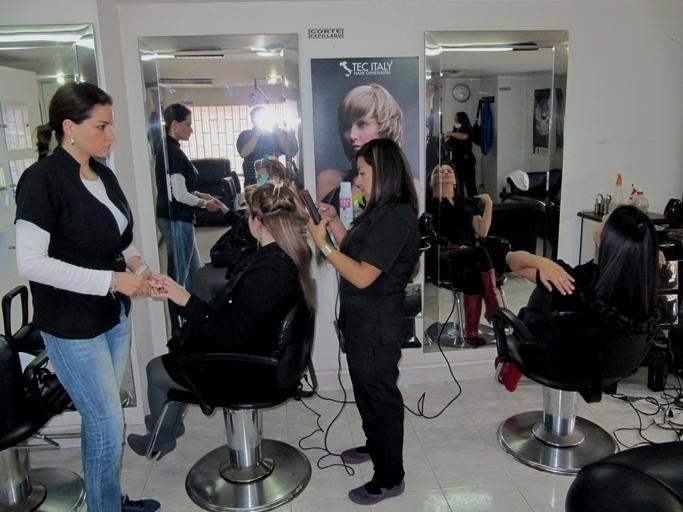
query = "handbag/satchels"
[472,120,484,145]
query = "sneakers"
[340,446,370,464]
[350,480,404,505]
[121,495,160,512]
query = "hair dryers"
[418,211,440,258]
[663,197,683,230]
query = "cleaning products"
[609,173,626,212]
[630,184,649,214]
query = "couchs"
[564,438,682,511]
[188,158,245,231]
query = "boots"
[495,358,521,391]
[464,294,485,347]
[483,267,501,325]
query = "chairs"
[499,168,554,237]
[424,215,511,345]
[145,296,318,512]
[490,294,652,474]
[0,283,88,511]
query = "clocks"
[451,83,470,102]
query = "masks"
[454,122,462,129]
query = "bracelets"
[133,264,148,275]
[110,271,117,294]
[319,243,335,256]
[199,199,207,209]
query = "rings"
[558,277,562,282]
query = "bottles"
[607,172,626,214]
[338,181,353,230]
[594,193,610,215]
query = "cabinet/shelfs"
[579,209,682,393]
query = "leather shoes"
[128,415,183,461]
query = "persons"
[426,162,499,350]
[191,157,288,306]
[441,112,479,204]
[494,204,660,391]
[234,106,296,187]
[154,103,219,323]
[126,181,315,461]
[13,83,161,512]
[313,83,422,284]
[308,138,423,506]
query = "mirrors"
[0,21,137,409]
[422,26,563,352]
[136,34,303,346]
